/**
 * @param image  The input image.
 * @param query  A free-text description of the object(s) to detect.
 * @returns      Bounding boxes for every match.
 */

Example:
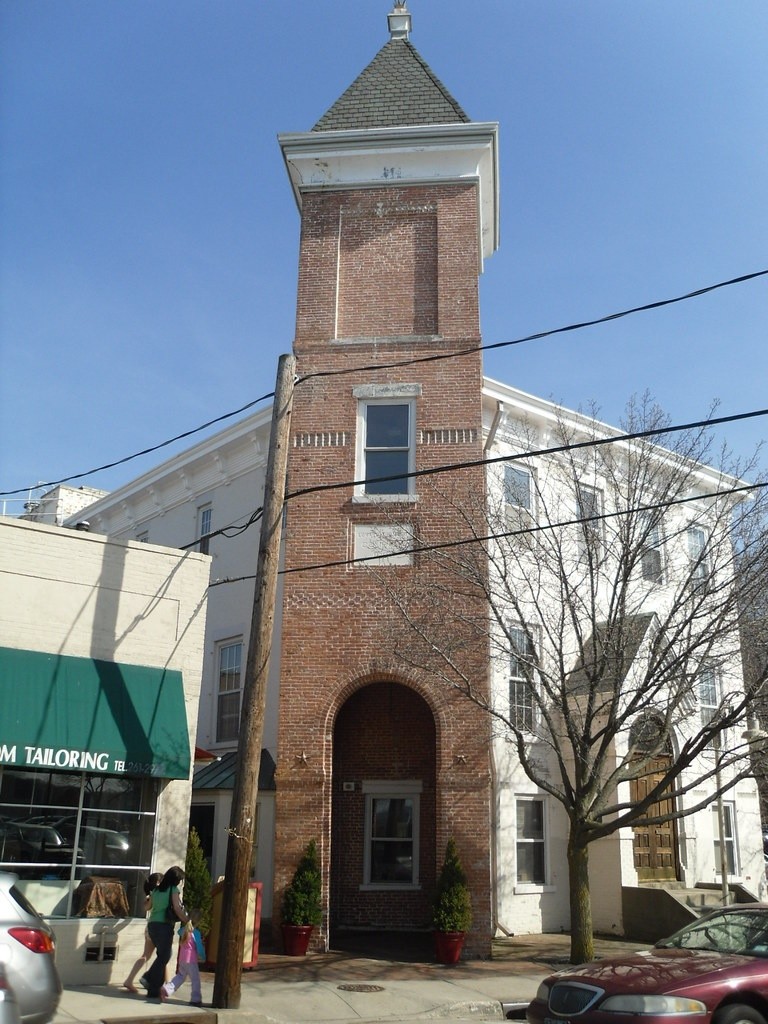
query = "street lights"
[709,688,767,954]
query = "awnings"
[0,644,191,782]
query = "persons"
[124,866,206,1008]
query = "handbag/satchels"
[166,886,188,921]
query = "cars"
[0,869,63,1024]
[525,899,768,1024]
[1,814,131,878]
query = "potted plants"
[176,826,215,974]
[430,835,474,964]
[279,838,323,957]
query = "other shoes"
[190,1002,204,1007]
[160,986,168,1003]
[140,976,149,991]
[124,982,139,993]
